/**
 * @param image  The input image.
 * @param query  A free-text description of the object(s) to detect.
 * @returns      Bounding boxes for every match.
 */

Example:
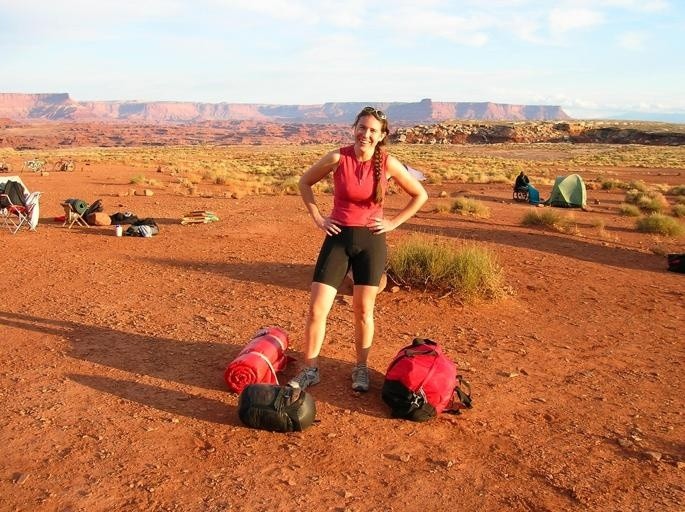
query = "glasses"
[361,106,389,123]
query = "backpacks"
[381,338,472,422]
[0,180,25,208]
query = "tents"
[544,173,588,208]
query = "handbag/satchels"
[126,223,158,237]
[110,213,137,225]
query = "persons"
[513,169,530,193]
[281,105,431,393]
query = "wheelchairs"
[513,179,541,203]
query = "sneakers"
[287,365,319,392]
[351,365,370,391]
